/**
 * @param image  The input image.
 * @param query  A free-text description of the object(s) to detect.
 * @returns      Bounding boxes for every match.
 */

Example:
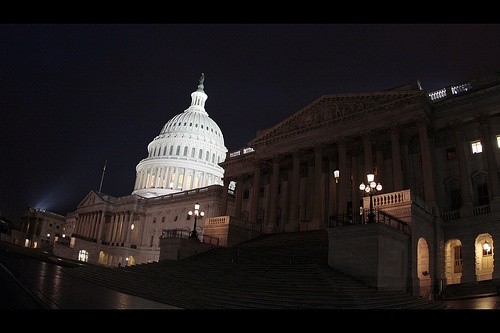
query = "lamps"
[334,169,340,183]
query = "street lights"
[187,202,204,240]
[358,172,383,223]
